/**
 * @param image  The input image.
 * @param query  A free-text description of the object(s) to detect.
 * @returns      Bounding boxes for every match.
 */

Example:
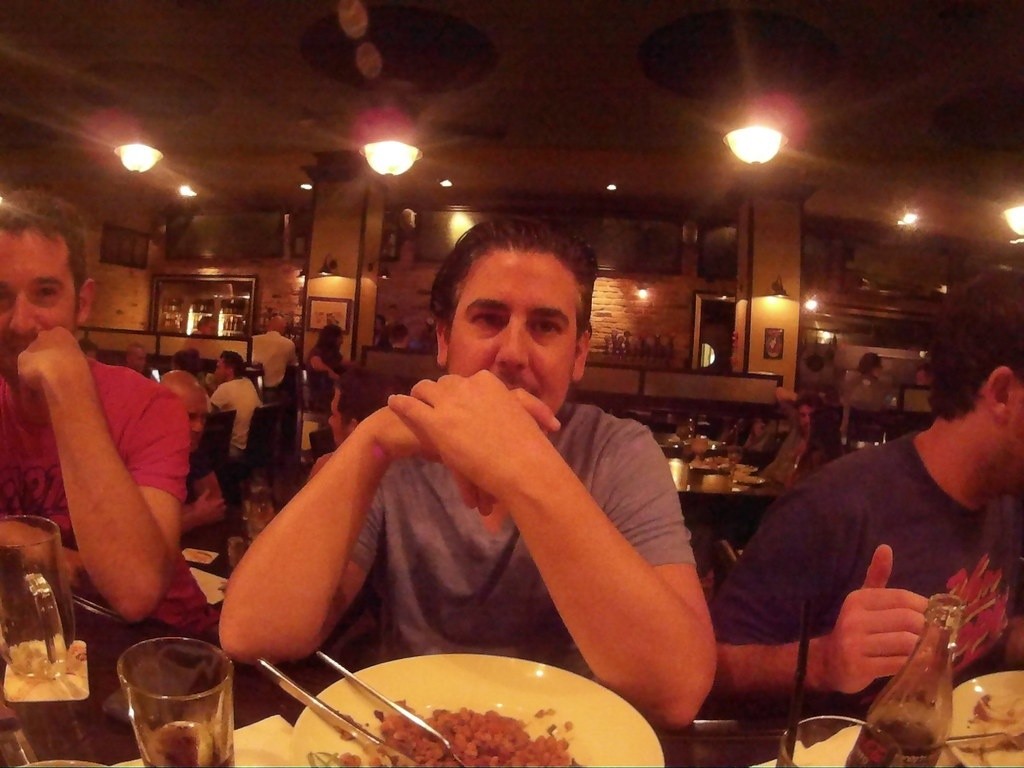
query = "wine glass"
[726,443,744,486]
[688,437,708,469]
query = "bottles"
[157,294,252,338]
[865,594,965,768]
[602,328,674,371]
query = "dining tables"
[668,456,778,595]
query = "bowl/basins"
[949,670,1024,768]
[290,653,665,767]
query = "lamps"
[112,135,164,172]
[352,100,423,176]
[296,270,306,278]
[377,263,391,281]
[318,254,338,277]
[765,279,792,299]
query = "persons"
[0,187,222,641]
[77,311,437,580]
[718,352,884,576]
[216,209,715,732]
[693,269,1024,720]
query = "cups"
[667,458,686,490]
[226,497,276,569]
[779,715,867,768]
[116,638,235,768]
[1,515,73,678]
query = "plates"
[689,457,766,486]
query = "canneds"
[225,314,242,332]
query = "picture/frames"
[763,328,785,360]
[306,296,352,334]
[99,224,151,270]
